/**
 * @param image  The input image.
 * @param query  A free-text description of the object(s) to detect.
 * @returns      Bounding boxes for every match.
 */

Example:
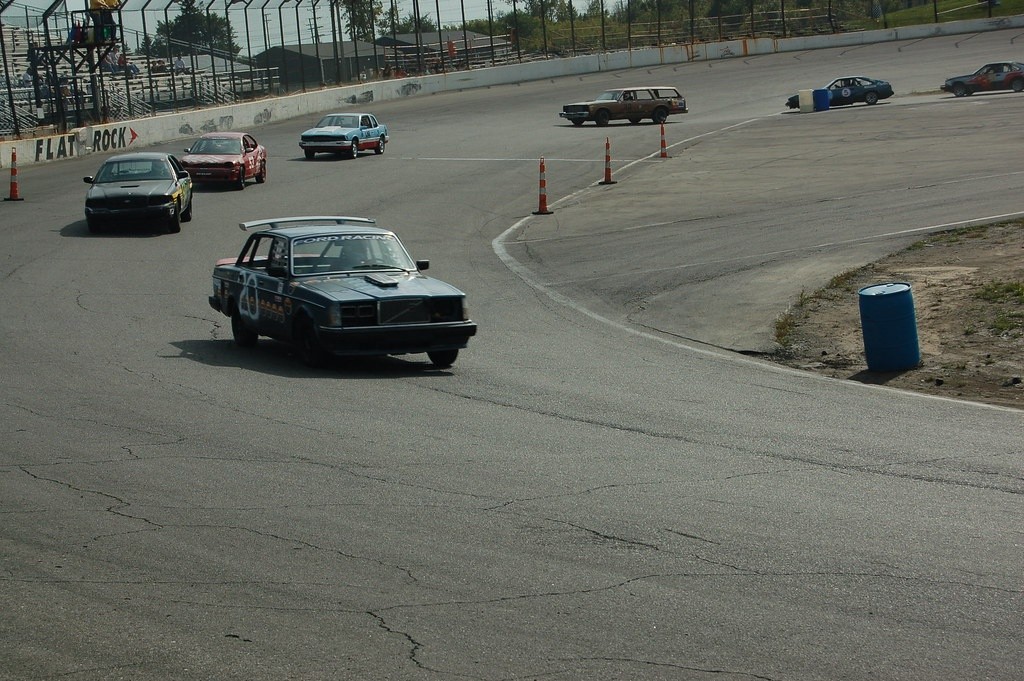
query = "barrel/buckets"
[857,282,920,373]
[798,89,814,113]
[814,88,830,111]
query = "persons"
[395,65,410,78]
[90,0,122,45]
[23,67,78,99]
[174,52,189,76]
[148,159,166,177]
[151,59,167,74]
[329,244,365,271]
[622,92,633,100]
[380,63,394,79]
[26,41,54,66]
[98,46,143,78]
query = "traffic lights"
[447,41,458,59]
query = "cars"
[181,133,268,190]
[83,150,194,234]
[941,61,1024,98]
[785,77,893,109]
[208,215,476,365]
[298,112,390,160]
[560,86,689,127]
